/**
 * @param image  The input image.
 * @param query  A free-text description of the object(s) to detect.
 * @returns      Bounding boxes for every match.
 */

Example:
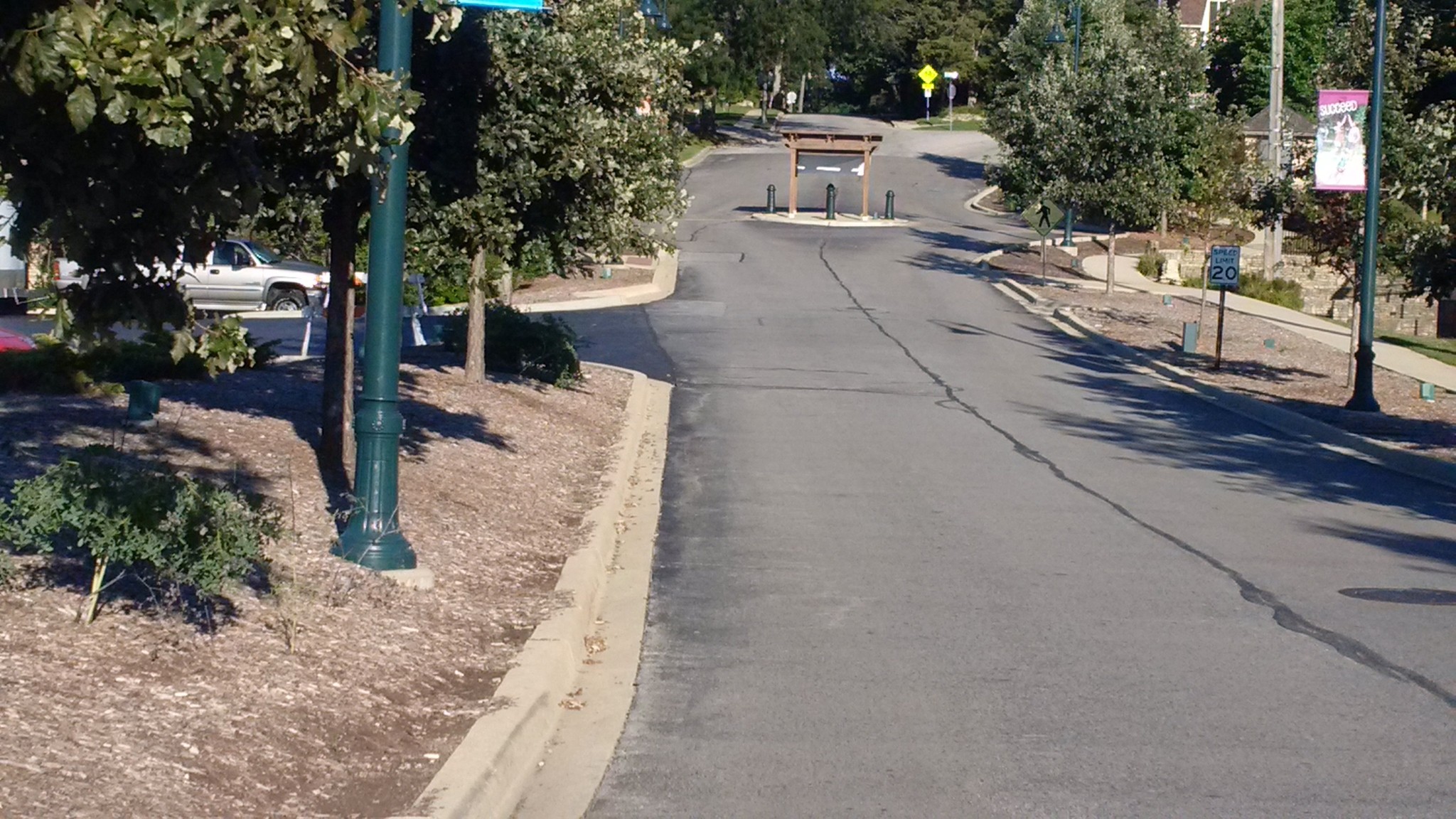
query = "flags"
[1313,89,1369,193]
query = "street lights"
[1046,1,1081,247]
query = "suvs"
[53,237,330,323]
